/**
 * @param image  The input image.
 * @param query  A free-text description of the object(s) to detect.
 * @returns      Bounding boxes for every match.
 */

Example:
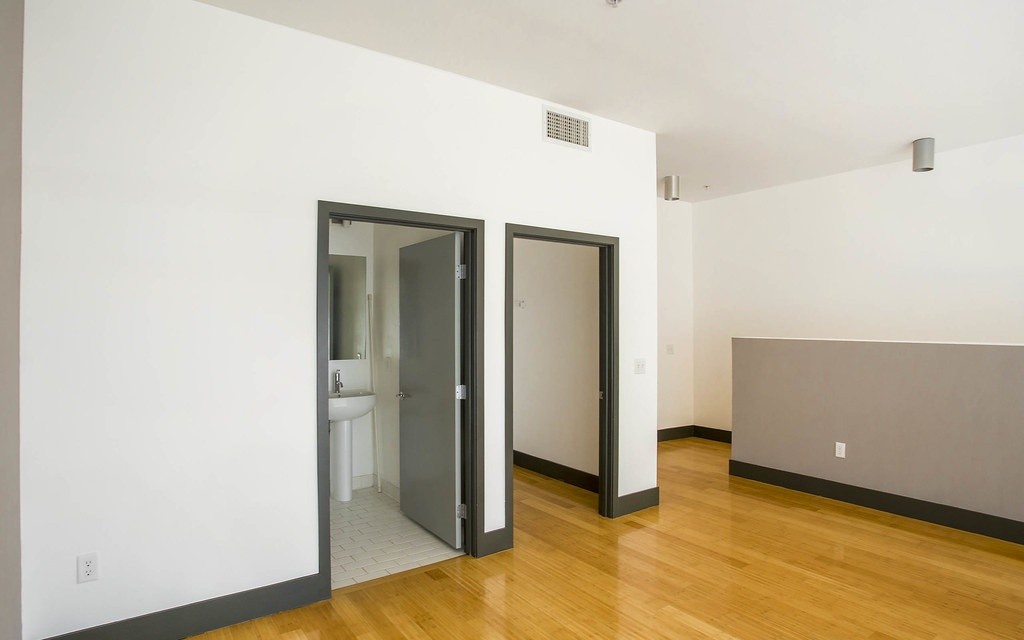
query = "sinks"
[328,389,377,422]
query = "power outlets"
[835,442,846,458]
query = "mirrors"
[328,255,366,360]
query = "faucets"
[334,369,343,394]
